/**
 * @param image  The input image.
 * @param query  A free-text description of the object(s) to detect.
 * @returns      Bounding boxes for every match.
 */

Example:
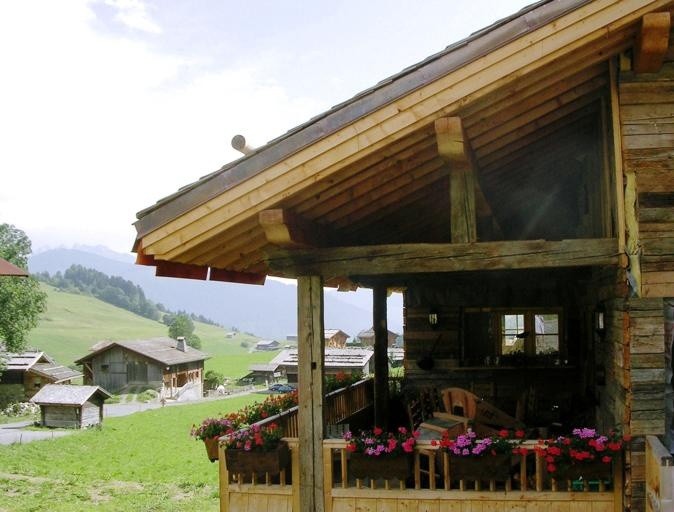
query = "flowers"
[533,428,632,470]
[191,388,300,440]
[319,370,365,394]
[342,425,421,455]
[431,430,528,456]
[216,423,277,451]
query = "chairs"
[406,382,527,443]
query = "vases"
[204,436,222,462]
[554,464,613,480]
[446,455,512,479]
